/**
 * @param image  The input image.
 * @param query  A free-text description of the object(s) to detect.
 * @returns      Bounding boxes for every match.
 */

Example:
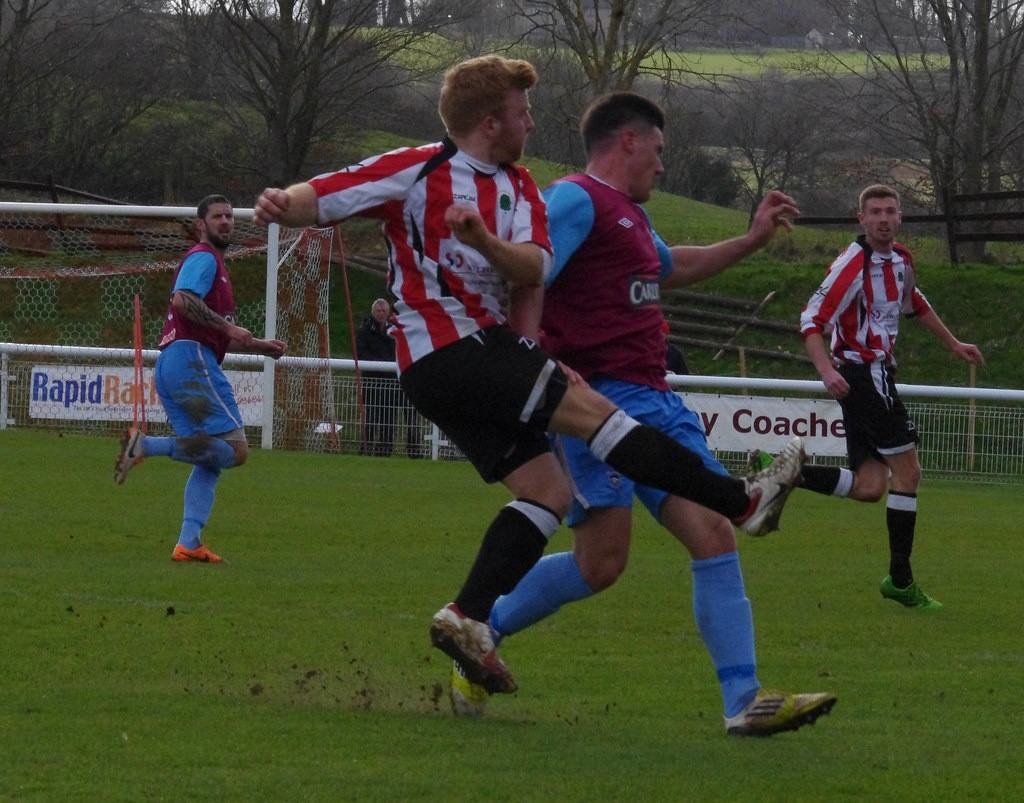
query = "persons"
[397,386,426,459]
[666,343,689,392]
[113,195,286,563]
[747,185,987,610]
[356,299,394,457]
[448,94,837,736]
[254,55,804,693]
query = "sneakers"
[746,449,776,477]
[171,543,223,564]
[113,427,146,484]
[738,437,804,537]
[722,688,837,739]
[448,621,504,719]
[430,602,519,694]
[880,575,944,609]
